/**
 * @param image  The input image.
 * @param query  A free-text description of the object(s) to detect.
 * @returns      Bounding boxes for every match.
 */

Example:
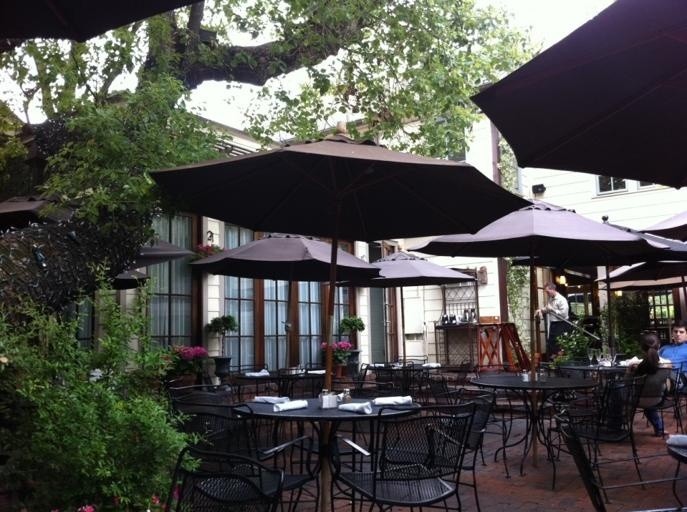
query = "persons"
[535,282,571,361]
[147,119,537,512]
[644,320,687,438]
[605,334,673,433]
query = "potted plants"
[205,315,239,378]
[340,317,365,375]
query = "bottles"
[539,369,546,383]
[343,388,352,404]
[464,309,475,322]
[522,368,527,382]
[321,389,329,409]
[441,314,448,324]
[449,314,456,324]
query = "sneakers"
[655,431,669,437]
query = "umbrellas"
[0,0,206,42]
[405,197,673,467]
[597,276,687,330]
[189,233,380,374]
[637,209,687,242]
[106,269,151,290]
[325,247,476,379]
[596,260,687,281]
[0,192,193,267]
[512,213,687,360]
[470,0,687,190]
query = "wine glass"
[587,348,601,368]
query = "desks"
[433,321,530,371]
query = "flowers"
[190,245,231,259]
[172,345,208,373]
[321,342,352,365]
